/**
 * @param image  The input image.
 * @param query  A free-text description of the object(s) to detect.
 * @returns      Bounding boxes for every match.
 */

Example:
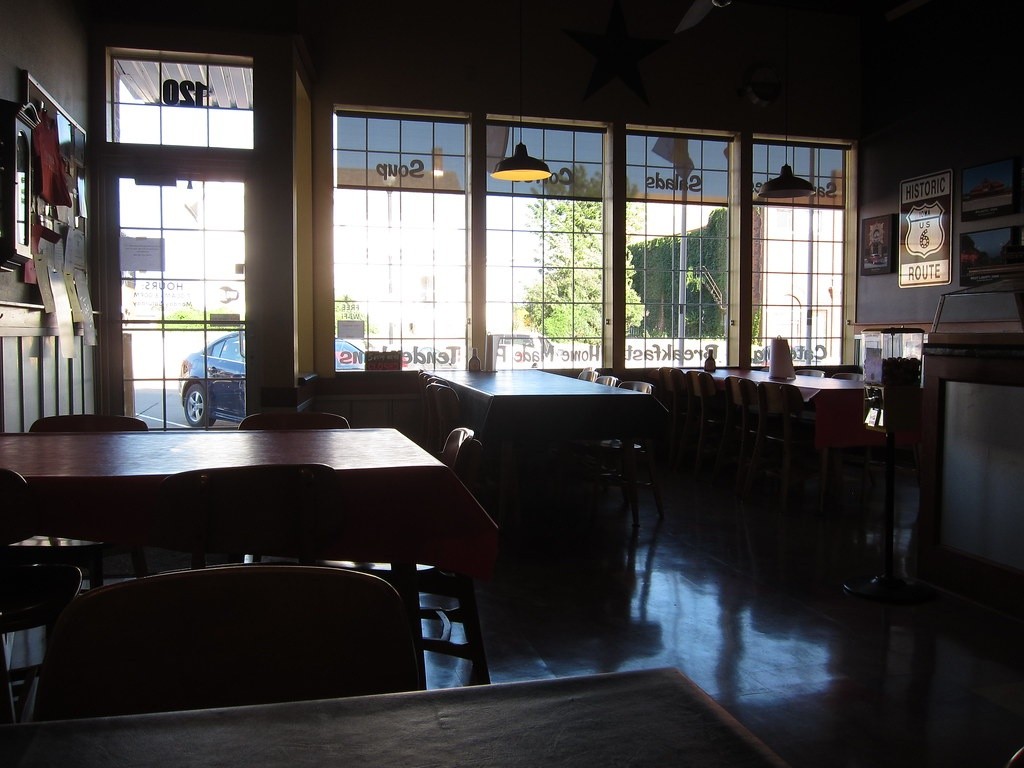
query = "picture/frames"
[858,214,899,278]
[958,156,1021,220]
[896,168,956,288]
[956,224,1024,291]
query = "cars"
[180,330,367,428]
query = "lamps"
[758,2,818,201]
[489,1,553,186]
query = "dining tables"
[1,367,874,766]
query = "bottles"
[704,349,717,373]
[468,348,481,372]
